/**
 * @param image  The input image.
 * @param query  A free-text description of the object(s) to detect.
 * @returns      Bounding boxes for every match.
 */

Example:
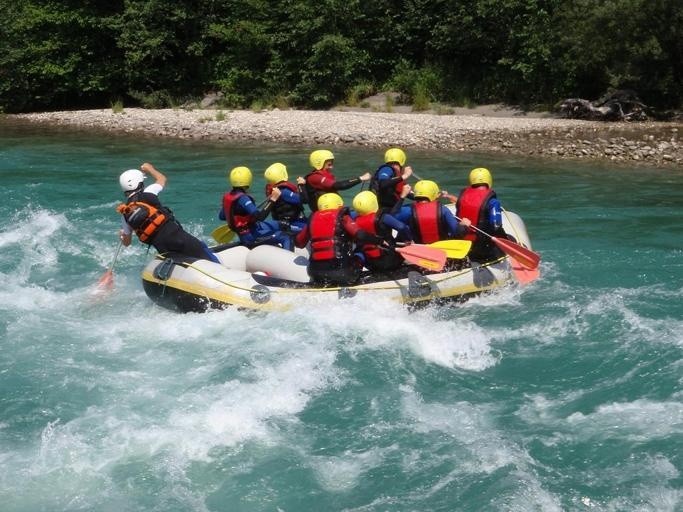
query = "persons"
[349,192,437,281]
[223,167,296,255]
[391,179,470,240]
[303,150,371,211]
[295,192,391,285]
[116,160,221,268]
[369,147,449,210]
[264,161,309,231]
[456,168,503,261]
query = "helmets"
[352,191,379,214]
[317,193,344,211]
[230,166,252,188]
[264,163,288,184]
[385,149,406,166]
[119,169,147,192]
[470,167,492,188]
[414,180,439,201]
[310,150,334,170]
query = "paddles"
[428,239,474,259]
[497,206,541,285]
[96,238,123,295]
[410,169,460,203]
[211,193,272,245]
[388,243,448,275]
[454,213,541,270]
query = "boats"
[142,202,538,311]
